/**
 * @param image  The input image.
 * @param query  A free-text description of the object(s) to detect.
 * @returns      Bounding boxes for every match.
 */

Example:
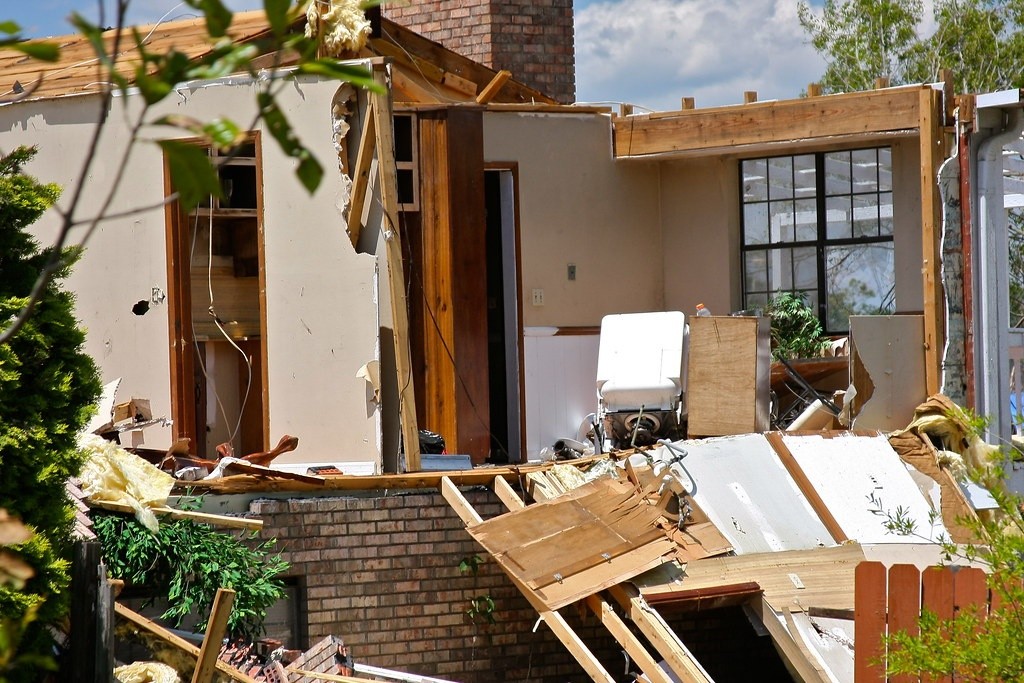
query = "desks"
[770,356,845,425]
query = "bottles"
[696,303,711,316]
